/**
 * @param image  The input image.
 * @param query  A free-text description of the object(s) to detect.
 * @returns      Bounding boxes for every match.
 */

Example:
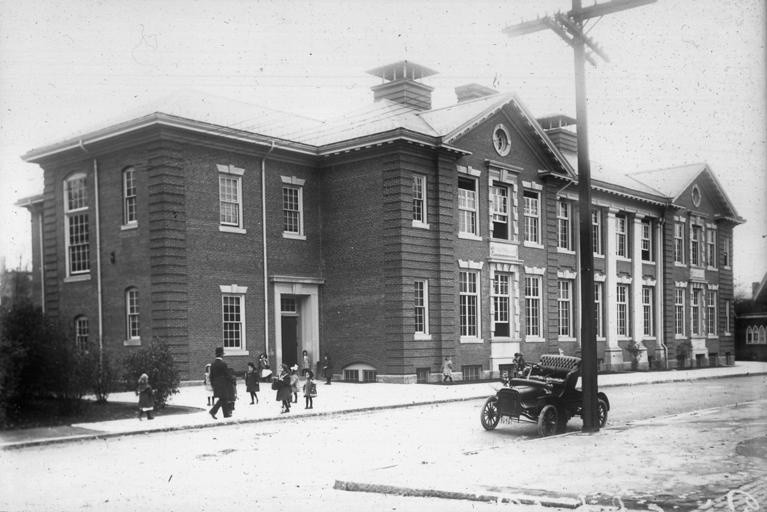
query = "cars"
[479,354,610,436]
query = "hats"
[214,346,226,355]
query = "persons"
[301,350,310,376]
[320,353,333,385]
[228,368,236,411]
[302,370,316,408]
[208,346,235,419]
[289,363,300,403]
[517,352,525,370]
[271,363,292,413]
[259,354,272,378]
[245,361,258,404]
[512,352,518,376]
[439,355,452,382]
[258,353,272,382]
[203,363,214,405]
[137,373,155,421]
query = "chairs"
[523,364,571,394]
